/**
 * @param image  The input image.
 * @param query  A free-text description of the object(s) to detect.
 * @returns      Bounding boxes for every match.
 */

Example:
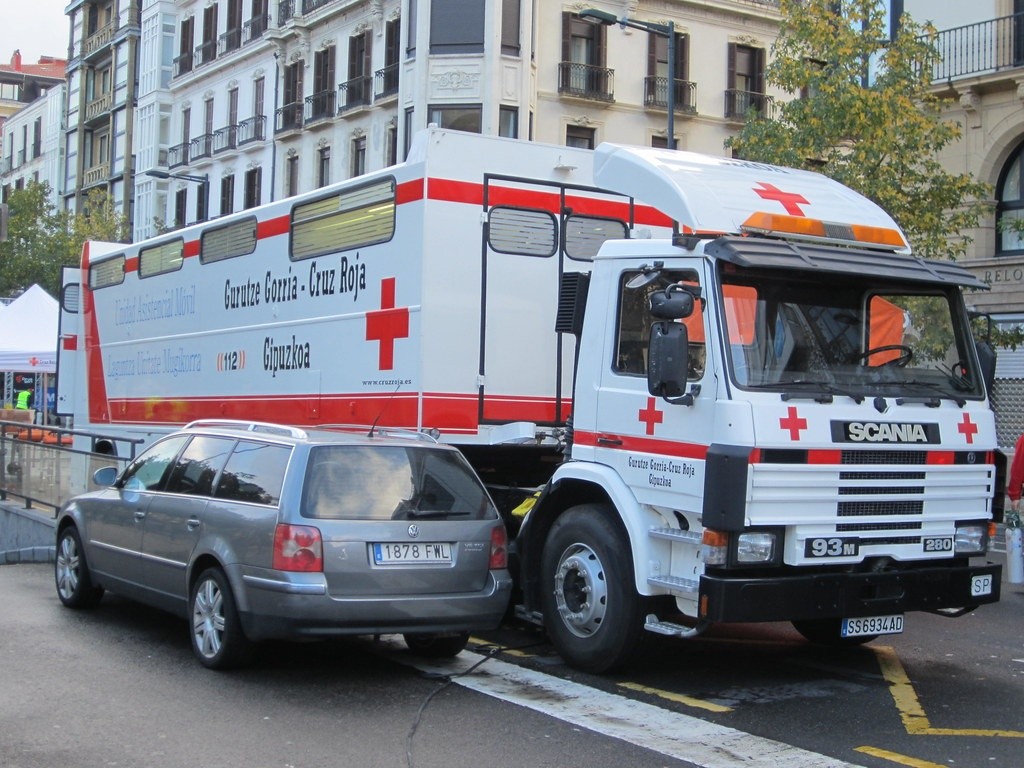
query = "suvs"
[55,419,519,674]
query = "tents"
[0,282,60,373]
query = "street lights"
[573,7,679,151]
[142,168,210,222]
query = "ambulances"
[55,120,1006,674]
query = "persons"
[1007,433,1024,512]
[16,385,35,409]
